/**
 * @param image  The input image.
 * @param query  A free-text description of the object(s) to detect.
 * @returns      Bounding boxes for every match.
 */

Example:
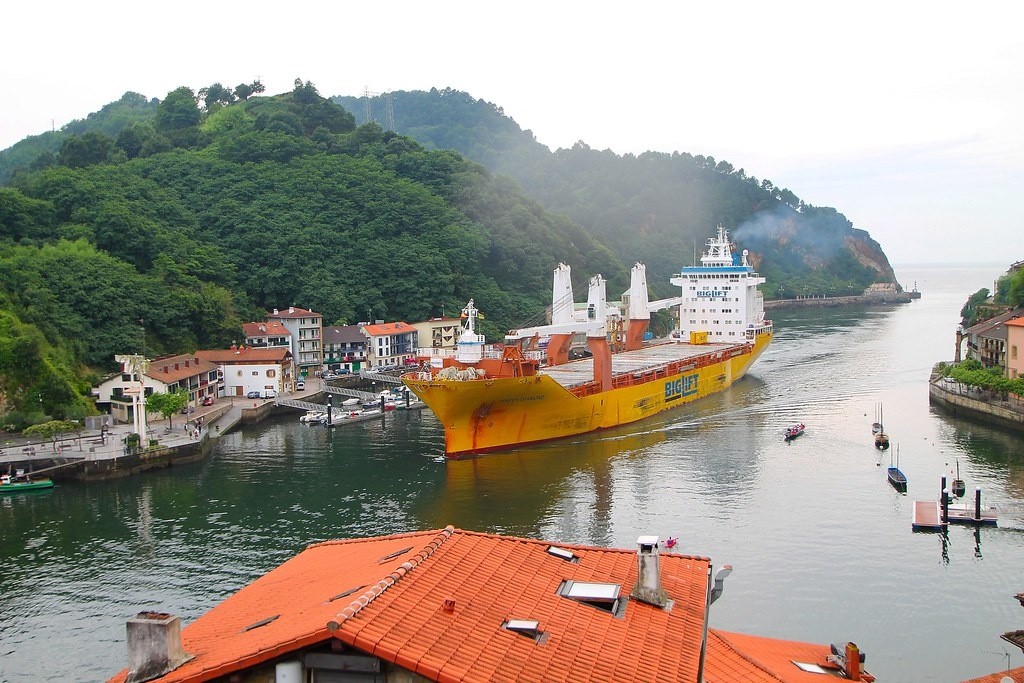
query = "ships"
[402,225,773,460]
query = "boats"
[784,422,805,441]
[665,538,676,548]
[0,475,54,493]
[299,385,424,425]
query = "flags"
[460,313,467,319]
[478,313,484,319]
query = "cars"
[182,405,194,413]
[318,368,349,381]
[202,397,214,406]
[992,392,1008,401]
[297,376,305,383]
[297,382,304,390]
[673,330,680,338]
[944,376,956,383]
[353,362,419,375]
[247,391,260,399]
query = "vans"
[259,389,278,399]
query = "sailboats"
[875,402,889,450]
[871,402,883,432]
[951,459,965,496]
[887,443,907,495]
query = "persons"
[790,423,805,435]
[123,445,128,455]
[184,424,199,440]
[667,536,673,544]
